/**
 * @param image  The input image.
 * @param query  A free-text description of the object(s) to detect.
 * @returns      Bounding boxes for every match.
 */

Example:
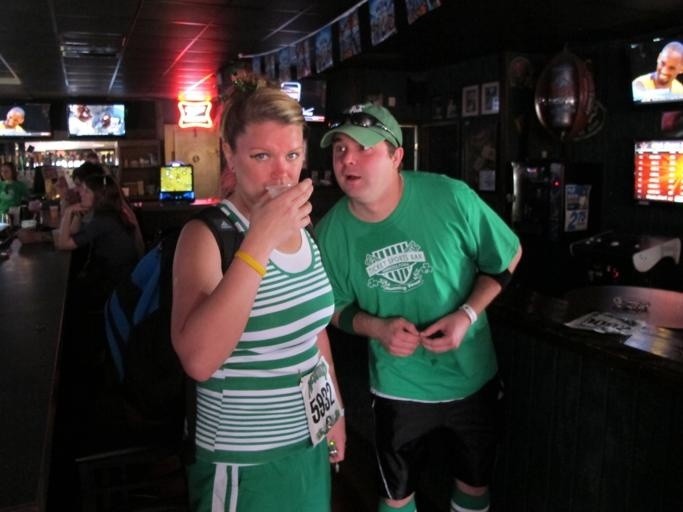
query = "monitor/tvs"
[65,102,127,140]
[0,102,53,140]
[624,32,683,114]
[627,136,683,207]
[158,164,195,202]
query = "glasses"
[327,113,401,147]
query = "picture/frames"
[480,80,499,115]
[460,83,479,118]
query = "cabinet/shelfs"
[1,136,164,220]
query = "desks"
[558,283,683,333]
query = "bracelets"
[459,304,478,329]
[234,252,266,277]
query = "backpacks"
[100,206,317,445]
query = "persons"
[314,102,522,512]
[170,77,348,512]
[633,41,683,99]
[0,107,25,135]
[1,147,145,324]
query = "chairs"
[70,375,200,512]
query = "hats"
[320,103,403,150]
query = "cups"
[262,182,299,199]
